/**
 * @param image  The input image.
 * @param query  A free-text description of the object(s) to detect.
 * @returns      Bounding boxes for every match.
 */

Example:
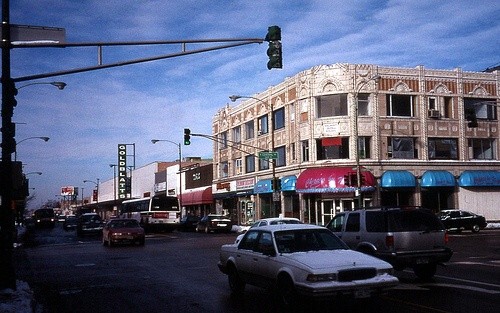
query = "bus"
[113,197,182,229]
[34,208,55,230]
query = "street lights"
[83,179,99,203]
[151,139,182,212]
[109,164,132,199]
[10,135,50,247]
[229,94,277,218]
[353,74,383,211]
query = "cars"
[197,214,233,233]
[438,209,488,233]
[235,217,304,245]
[217,223,399,302]
[103,218,146,246]
[57,214,77,228]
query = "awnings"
[280,175,297,192]
[177,187,213,206]
[421,170,457,187]
[379,170,416,187]
[253,178,280,194]
[458,170,500,187]
[295,166,377,193]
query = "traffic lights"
[184,128,190,145]
[344,174,366,188]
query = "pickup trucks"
[325,206,454,269]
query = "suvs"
[77,213,103,236]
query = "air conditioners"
[428,110,440,117]
